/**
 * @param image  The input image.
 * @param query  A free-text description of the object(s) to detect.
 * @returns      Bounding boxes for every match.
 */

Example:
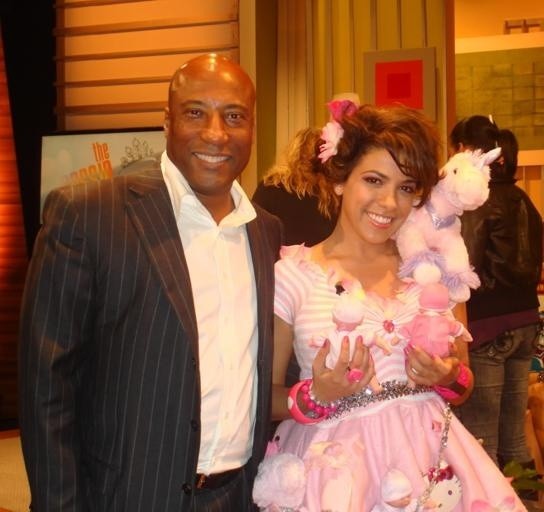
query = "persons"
[253,100,529,512]
[22,53,300,512]
[450,114,544,502]
[253,126,340,247]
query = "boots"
[513,459,539,502]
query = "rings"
[347,366,363,383]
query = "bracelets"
[302,379,336,415]
[436,364,470,400]
[308,380,341,408]
[287,382,327,425]
